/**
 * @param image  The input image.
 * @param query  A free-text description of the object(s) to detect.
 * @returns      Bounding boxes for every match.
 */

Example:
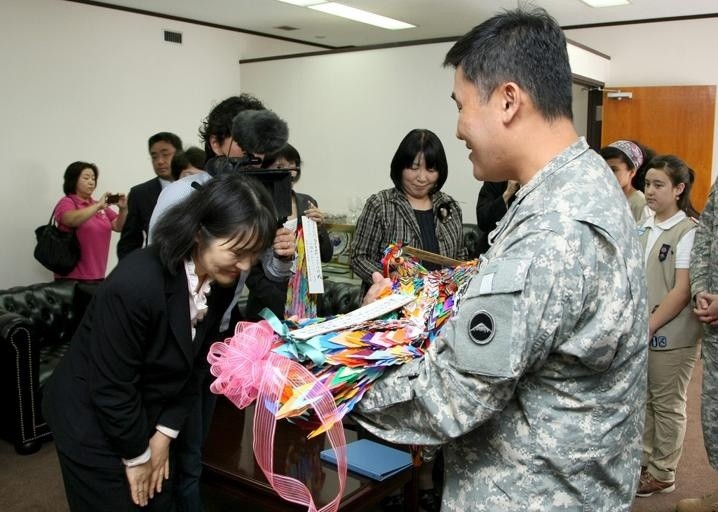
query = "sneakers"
[675,489,718,512]
[636,466,675,497]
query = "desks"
[201,384,423,512]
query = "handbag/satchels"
[34,225,81,276]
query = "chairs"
[0,279,101,455]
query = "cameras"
[105,194,120,203]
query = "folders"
[320,438,413,481]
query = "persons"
[599,139,717,511]
[331,2,647,512]
[40,93,522,512]
